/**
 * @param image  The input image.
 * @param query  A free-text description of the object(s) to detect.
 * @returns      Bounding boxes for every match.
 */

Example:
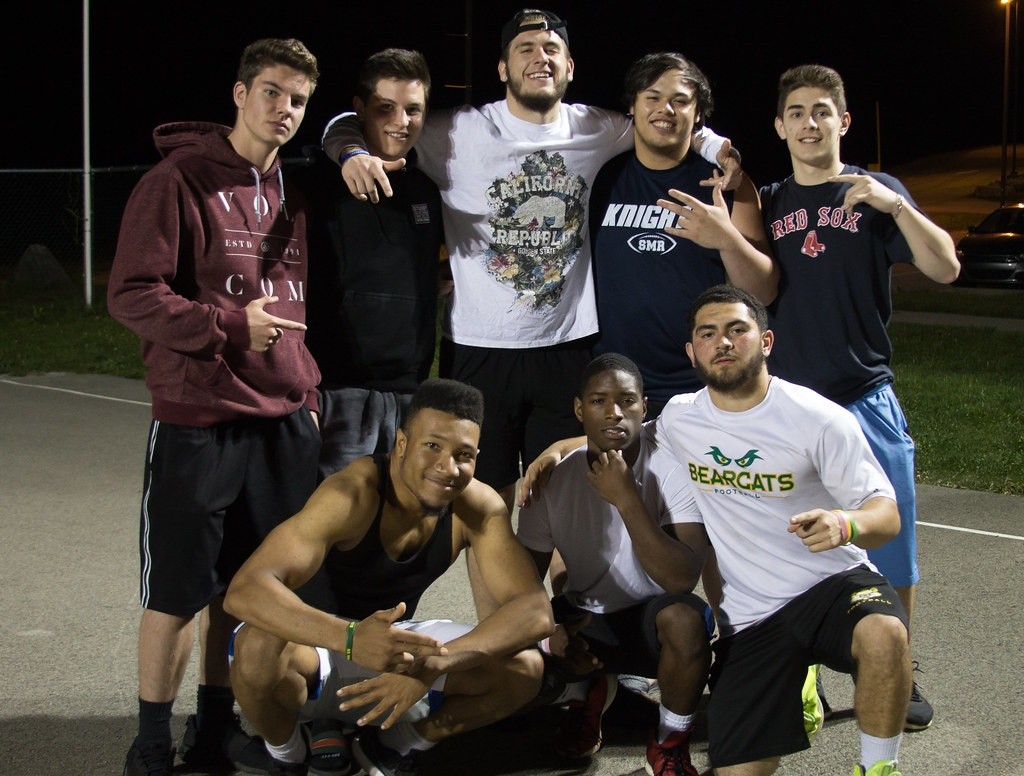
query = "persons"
[766,65,959,732]
[514,353,710,776]
[586,53,780,414]
[219,377,554,776]
[319,6,743,658]
[515,284,911,776]
[104,40,324,776]
[310,48,452,775]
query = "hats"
[501,8,569,49]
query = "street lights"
[1000,0,1013,206]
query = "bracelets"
[894,194,903,218]
[343,150,369,160]
[833,510,857,546]
[345,622,357,662]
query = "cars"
[950,203,1024,290]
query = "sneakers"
[181,713,269,775]
[269,729,311,776]
[552,671,619,757]
[353,728,427,776]
[802,665,833,738]
[645,726,699,776]
[121,736,178,776]
[853,760,902,776]
[902,660,934,731]
